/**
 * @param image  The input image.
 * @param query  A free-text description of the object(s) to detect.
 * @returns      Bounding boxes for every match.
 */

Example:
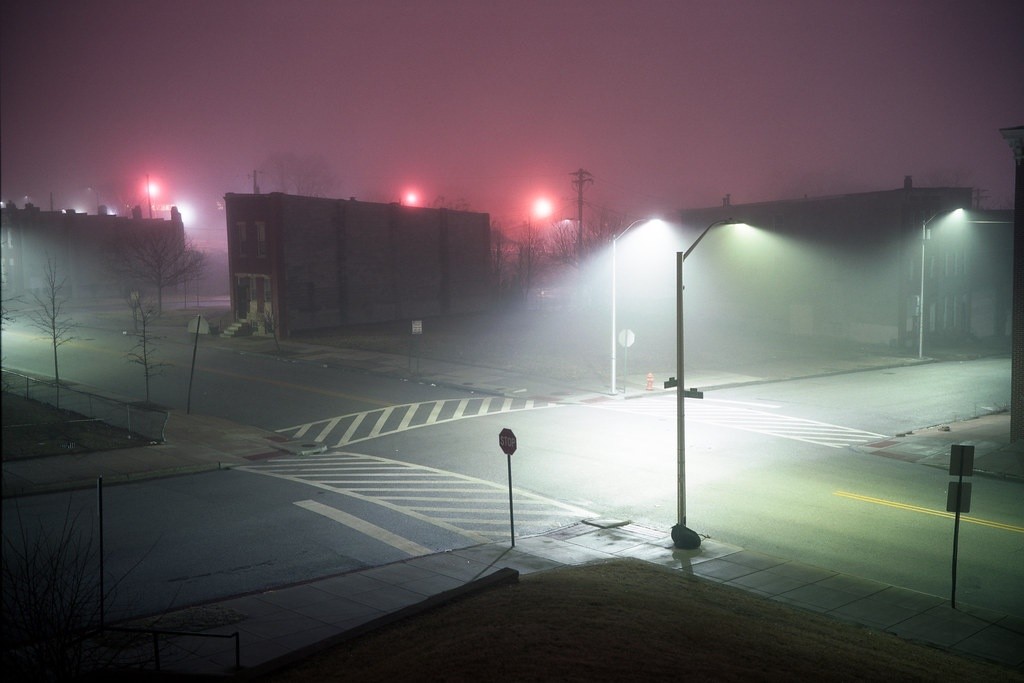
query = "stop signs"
[499,428,518,456]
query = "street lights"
[610,217,663,395]
[917,207,965,359]
[663,217,747,547]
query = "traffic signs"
[412,320,422,335]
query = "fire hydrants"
[645,372,655,391]
